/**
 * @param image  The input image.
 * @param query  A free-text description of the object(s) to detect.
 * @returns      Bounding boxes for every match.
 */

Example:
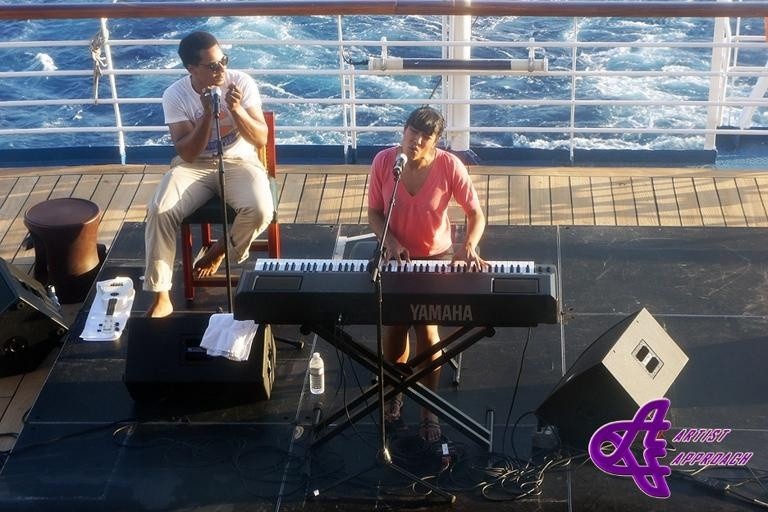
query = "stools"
[366,333,474,388]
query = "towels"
[200,309,259,363]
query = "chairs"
[179,106,283,302]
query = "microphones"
[211,87,221,118]
[392,153,408,176]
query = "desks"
[18,196,101,306]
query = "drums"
[24,197,102,288]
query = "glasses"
[196,54,229,72]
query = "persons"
[142,32,274,319]
[368,107,489,444]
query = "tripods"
[215,121,304,350]
[306,272,458,504]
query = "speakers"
[0,258,69,378]
[125,318,277,403]
[535,307,689,441]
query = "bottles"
[47,285,58,304]
[307,351,324,395]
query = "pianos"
[233,258,559,324]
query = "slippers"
[419,419,441,443]
[384,398,404,423]
